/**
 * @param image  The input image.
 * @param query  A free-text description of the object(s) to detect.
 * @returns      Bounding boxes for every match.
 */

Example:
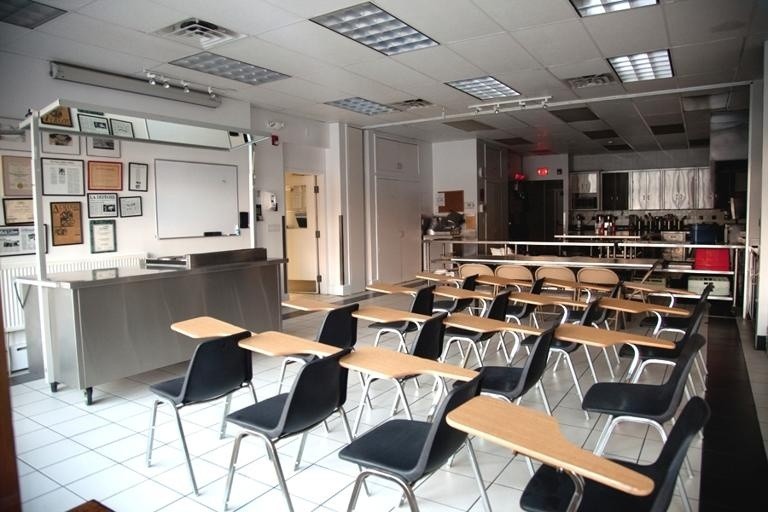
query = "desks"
[13,257,290,409]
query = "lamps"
[466,94,552,116]
[143,68,235,99]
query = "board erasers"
[204,232,222,236]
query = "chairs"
[144,263,715,512]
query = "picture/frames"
[92,268,118,281]
[0,106,148,254]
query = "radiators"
[0,251,149,335]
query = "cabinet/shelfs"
[362,128,421,180]
[629,169,662,211]
[663,168,696,210]
[422,234,463,271]
[449,232,744,315]
[600,170,629,210]
[364,174,422,290]
[696,167,714,209]
[568,172,600,193]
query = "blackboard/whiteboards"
[154,158,240,240]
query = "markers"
[229,234,237,235]
